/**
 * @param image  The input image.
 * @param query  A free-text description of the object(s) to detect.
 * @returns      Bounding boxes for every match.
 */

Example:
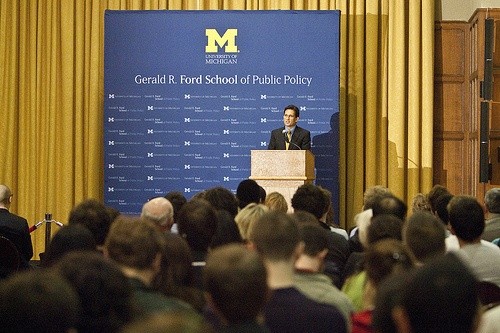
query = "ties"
[285,129,291,150]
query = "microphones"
[282,129,288,138]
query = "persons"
[447,196,500,288]
[0,184,33,261]
[482,188,500,240]
[268,105,311,151]
[177,180,351,333]
[0,192,208,333]
[341,185,480,333]
